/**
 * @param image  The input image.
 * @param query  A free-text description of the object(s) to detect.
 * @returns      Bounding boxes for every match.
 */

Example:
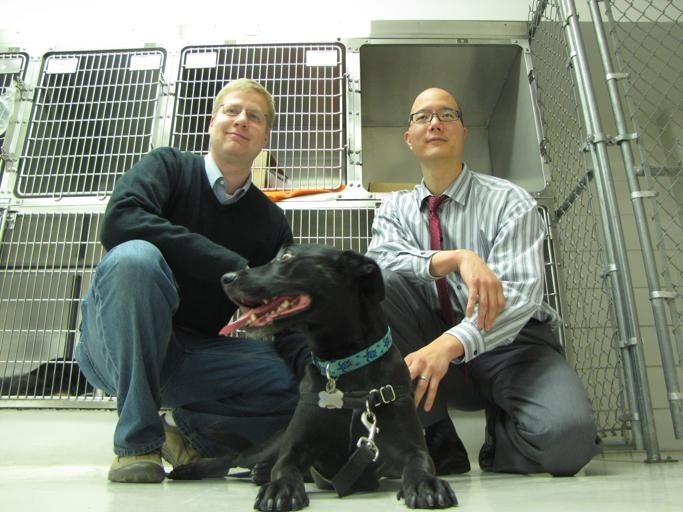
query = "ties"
[427,195,454,323]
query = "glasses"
[410,111,458,121]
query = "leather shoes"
[425,418,495,475]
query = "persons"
[363,88,598,478]
[68,80,308,487]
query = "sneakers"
[108,413,229,482]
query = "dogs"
[217,242,458,512]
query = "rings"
[418,375,429,383]
